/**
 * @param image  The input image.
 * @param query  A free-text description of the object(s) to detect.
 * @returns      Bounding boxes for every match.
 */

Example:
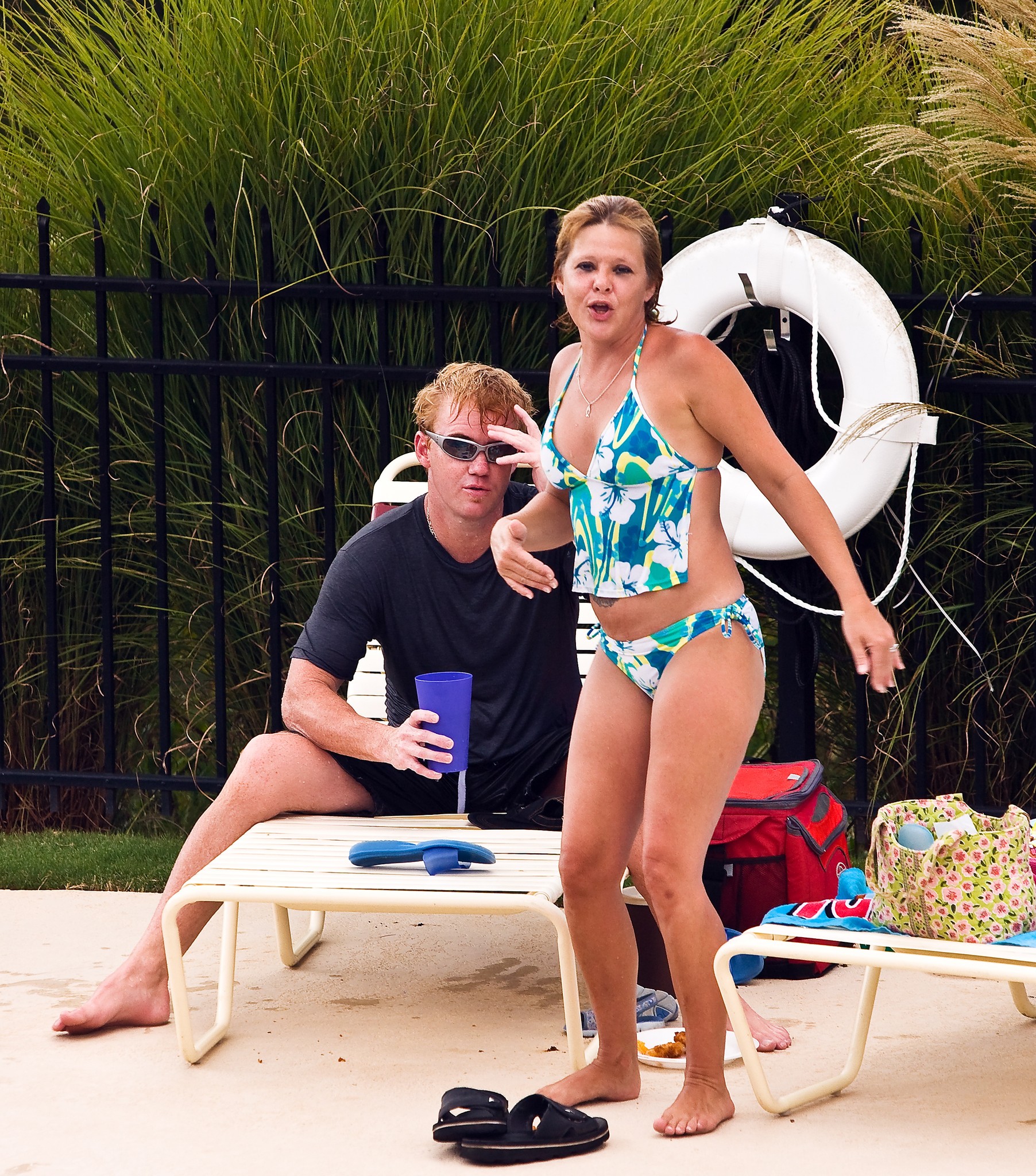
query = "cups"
[415,672,473,774]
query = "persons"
[490,191,906,1140]
[51,362,793,1053]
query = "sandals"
[563,992,666,1037]
[635,986,678,1020]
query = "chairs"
[159,452,632,1072]
[712,864,1036,1117]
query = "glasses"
[421,427,518,463]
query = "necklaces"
[577,344,640,418]
[425,496,440,544]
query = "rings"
[889,643,900,653]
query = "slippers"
[431,1086,508,1141]
[461,1095,609,1164]
[349,838,496,874]
[722,927,764,985]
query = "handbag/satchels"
[863,790,1035,943]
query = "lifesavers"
[653,224,920,561]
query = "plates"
[634,1027,760,1069]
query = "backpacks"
[713,757,849,978]
